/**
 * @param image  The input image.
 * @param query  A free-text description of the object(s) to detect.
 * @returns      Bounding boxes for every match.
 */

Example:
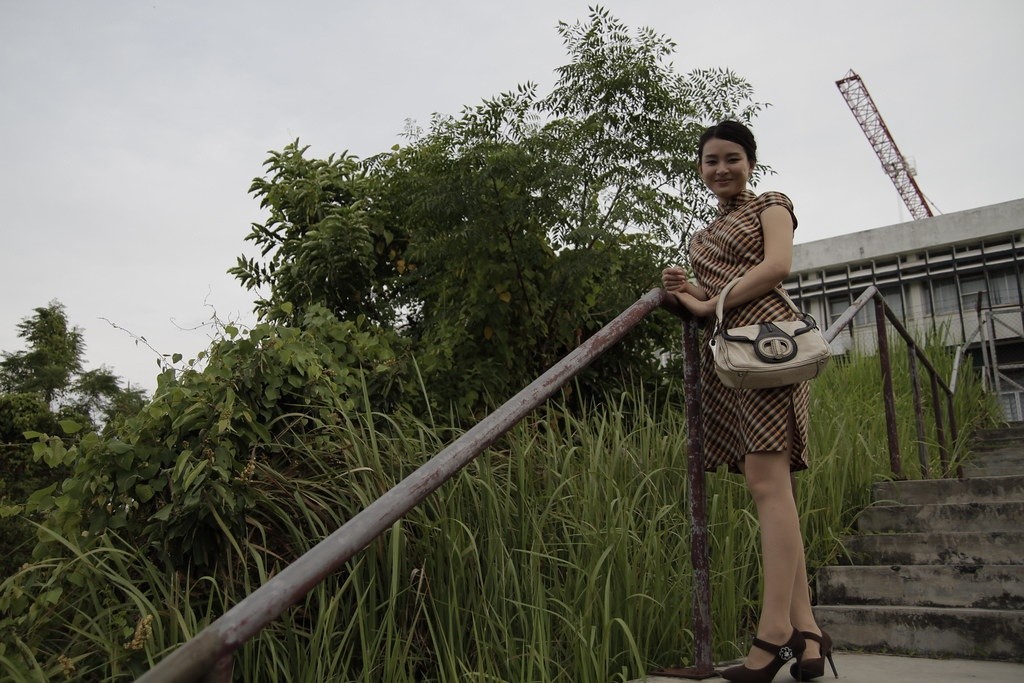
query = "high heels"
[720,626,806,682]
[790,627,839,682]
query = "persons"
[660,120,839,683]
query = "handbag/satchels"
[707,277,834,389]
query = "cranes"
[835,68,933,221]
